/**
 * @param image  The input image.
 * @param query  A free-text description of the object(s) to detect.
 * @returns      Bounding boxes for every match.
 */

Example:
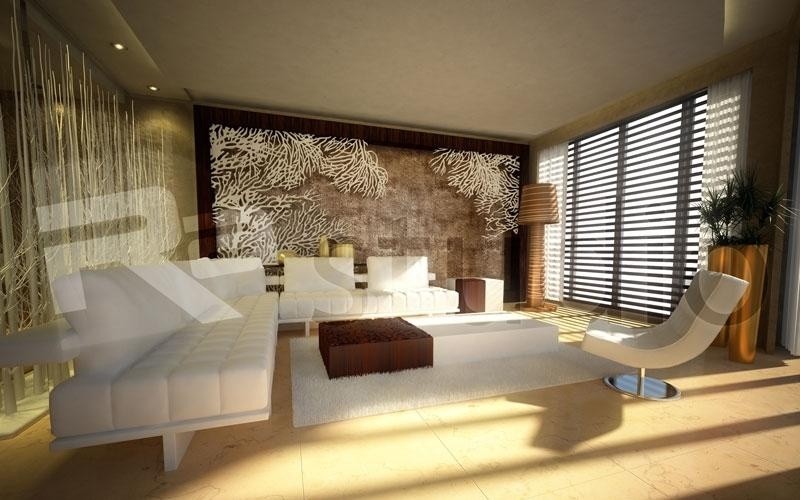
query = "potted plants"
[699,165,799,365]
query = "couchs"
[3,256,279,473]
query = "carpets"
[288,336,639,428]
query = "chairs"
[582,270,749,400]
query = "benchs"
[280,256,460,336]
